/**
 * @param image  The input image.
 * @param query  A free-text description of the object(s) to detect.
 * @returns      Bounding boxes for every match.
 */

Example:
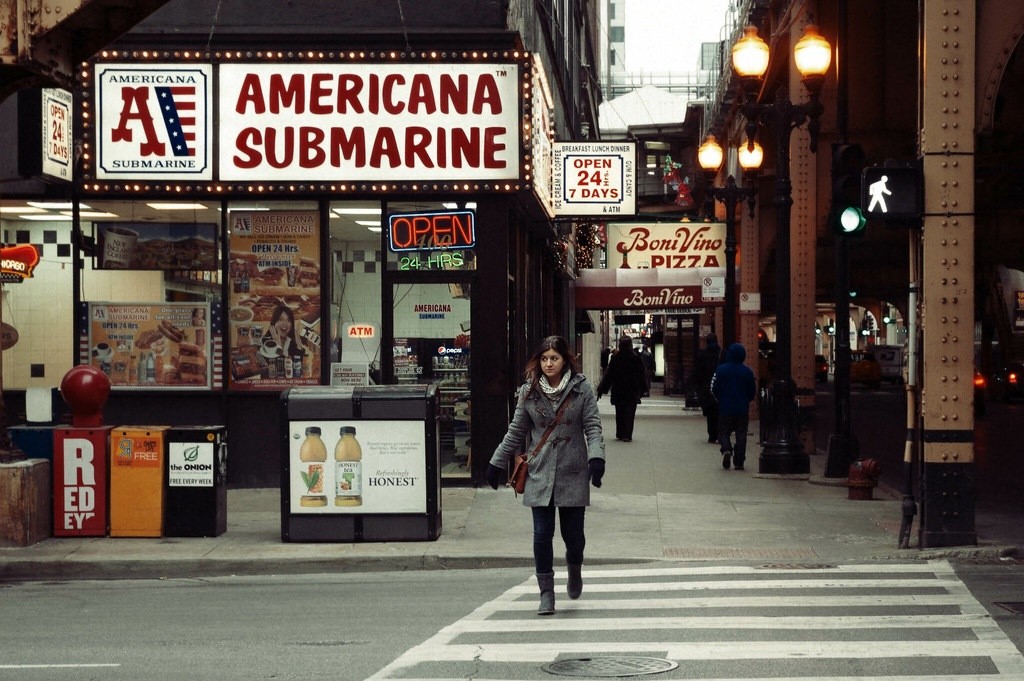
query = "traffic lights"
[860,162,924,220]
[832,145,873,242]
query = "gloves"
[487,463,502,489]
[587,457,605,488]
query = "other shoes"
[722,451,731,469]
[735,466,745,470]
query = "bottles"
[432,372,467,388]
[299,427,329,507]
[302,354,311,378]
[128,351,157,386]
[293,356,301,377]
[334,426,363,507]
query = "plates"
[260,345,283,359]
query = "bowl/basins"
[228,306,254,327]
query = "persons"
[261,307,303,358]
[595,333,656,442]
[488,335,606,615]
[192,308,207,327]
[710,342,756,471]
[692,333,724,442]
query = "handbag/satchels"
[509,454,527,494]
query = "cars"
[811,354,829,385]
[843,344,1018,411]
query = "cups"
[102,228,139,269]
[264,340,282,354]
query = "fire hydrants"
[843,447,884,504]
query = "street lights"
[696,131,765,366]
[732,23,835,476]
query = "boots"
[535,572,555,615]
[565,562,584,599]
